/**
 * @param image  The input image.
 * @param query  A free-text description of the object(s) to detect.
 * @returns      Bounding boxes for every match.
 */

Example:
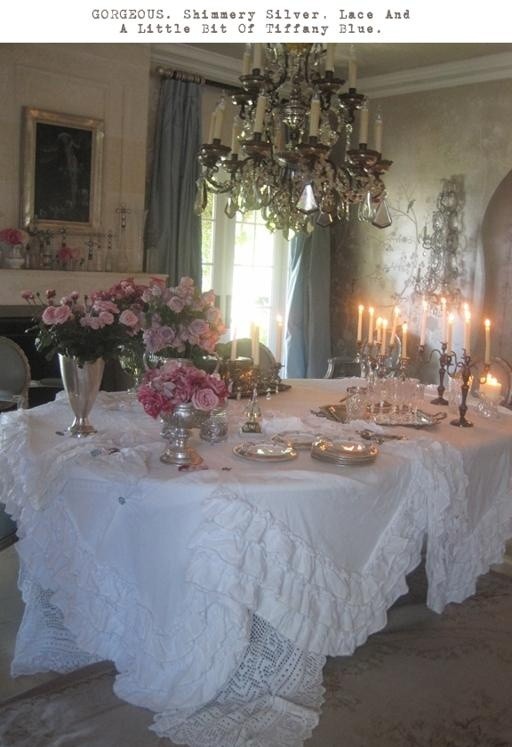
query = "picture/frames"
[1,376,512,661]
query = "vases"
[1,226,31,247]
[23,275,235,418]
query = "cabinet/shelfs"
[29,228,131,271]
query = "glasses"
[192,44,396,234]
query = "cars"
[477,390,505,421]
[345,386,370,415]
[449,372,473,410]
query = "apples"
[356,283,493,364]
[228,318,283,367]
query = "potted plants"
[17,105,105,233]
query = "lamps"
[457,355,511,406]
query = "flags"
[158,397,209,466]
[6,248,24,269]
[51,351,106,437]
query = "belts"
[373,376,426,424]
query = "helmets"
[309,435,378,468]
[272,430,316,450]
[232,439,300,462]
